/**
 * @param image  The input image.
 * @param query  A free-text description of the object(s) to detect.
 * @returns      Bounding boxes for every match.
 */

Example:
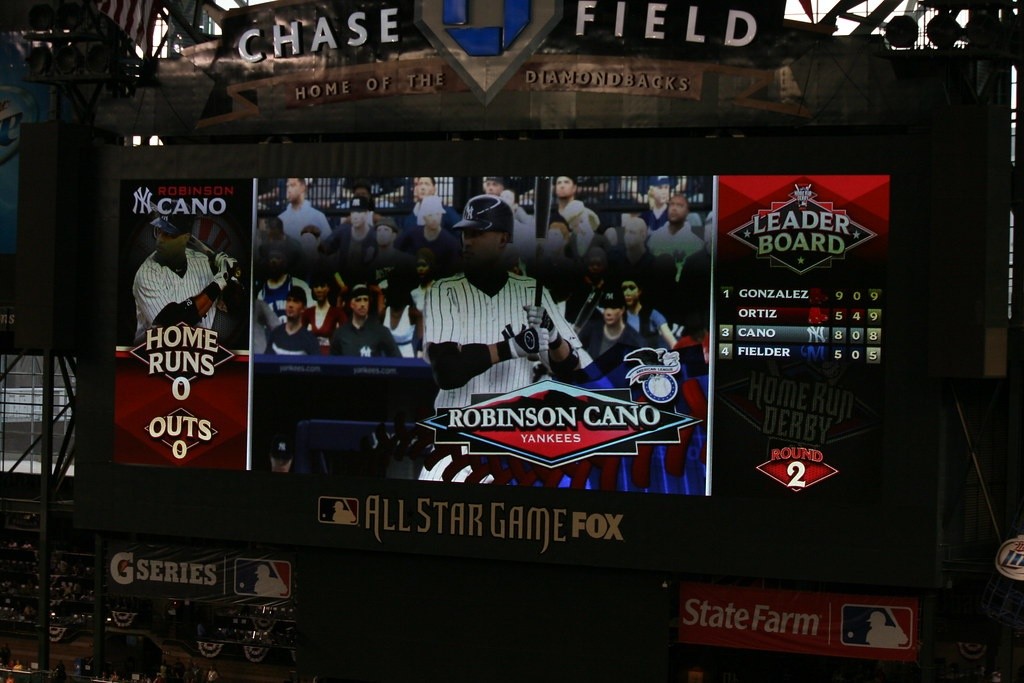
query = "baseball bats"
[188,234,235,276]
[526,175,555,364]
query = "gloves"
[213,250,238,289]
[523,303,563,349]
[497,325,549,363]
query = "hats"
[150,201,194,236]
[586,246,607,266]
[649,176,669,187]
[414,247,437,269]
[598,288,625,310]
[348,196,369,212]
[346,283,370,303]
[452,195,515,235]
[288,284,308,301]
[265,239,293,264]
[375,216,400,233]
[269,432,293,460]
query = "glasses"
[622,285,636,290]
[415,262,429,268]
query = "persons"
[132,200,241,351]
[420,194,583,480]
[256,176,709,371]
